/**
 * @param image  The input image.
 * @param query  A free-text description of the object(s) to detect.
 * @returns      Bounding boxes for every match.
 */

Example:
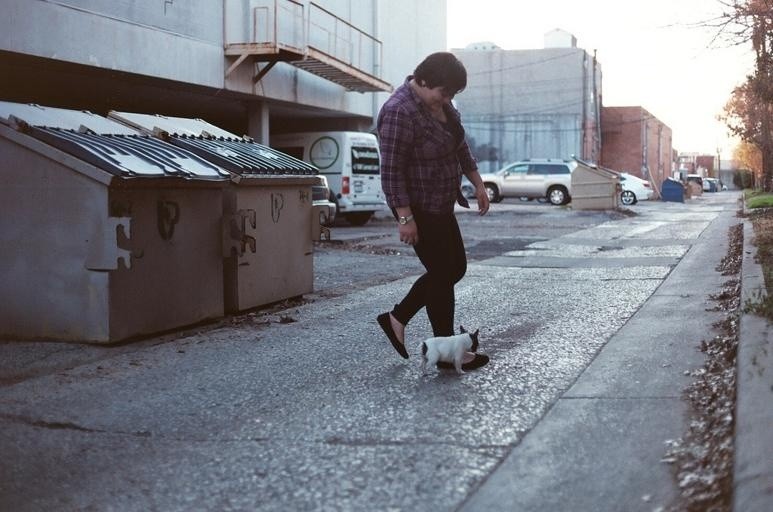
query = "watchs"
[398,215,414,225]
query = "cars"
[310,175,337,227]
[460,180,534,202]
[618,171,654,205]
[686,174,728,192]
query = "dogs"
[416,324,481,376]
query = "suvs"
[472,159,577,206]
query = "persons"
[377,52,490,370]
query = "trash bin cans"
[0,100,232,344]
[105,110,323,315]
[661,177,684,202]
[571,154,620,210]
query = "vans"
[271,132,390,226]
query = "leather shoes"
[376,312,410,359]
[437,353,489,372]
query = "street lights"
[715,146,723,180]
